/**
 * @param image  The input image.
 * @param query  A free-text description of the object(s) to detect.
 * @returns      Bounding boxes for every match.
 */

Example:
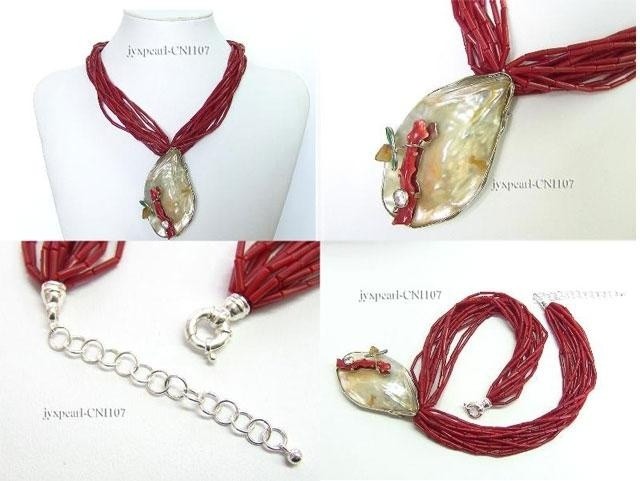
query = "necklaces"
[86,38,247,240]
[376,0,636,229]
[19,241,319,465]
[335,291,598,457]
